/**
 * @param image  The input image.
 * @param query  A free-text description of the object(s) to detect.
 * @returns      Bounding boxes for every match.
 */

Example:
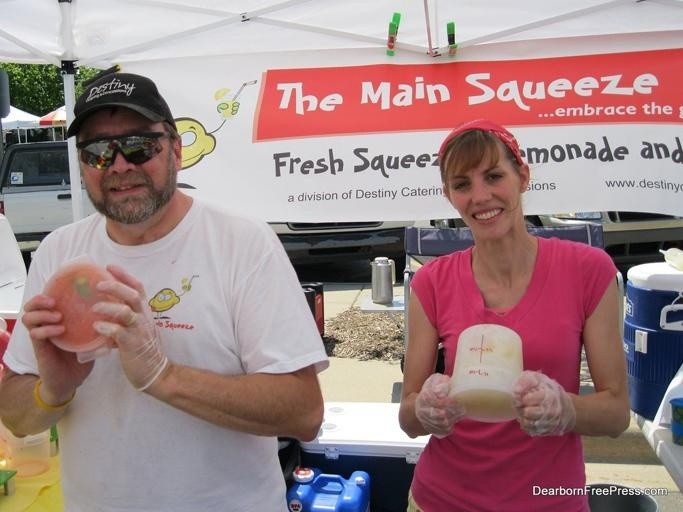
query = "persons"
[0,74,328,512]
[399,121,631,512]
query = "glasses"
[76,131,171,171]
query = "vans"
[0,140,95,241]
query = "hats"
[67,74,177,137]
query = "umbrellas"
[39,103,66,141]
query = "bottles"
[370,258,397,302]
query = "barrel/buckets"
[620,261,682,420]
[445,325,526,422]
[287,466,371,512]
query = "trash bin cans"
[301,282,325,337]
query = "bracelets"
[32,376,79,412]
